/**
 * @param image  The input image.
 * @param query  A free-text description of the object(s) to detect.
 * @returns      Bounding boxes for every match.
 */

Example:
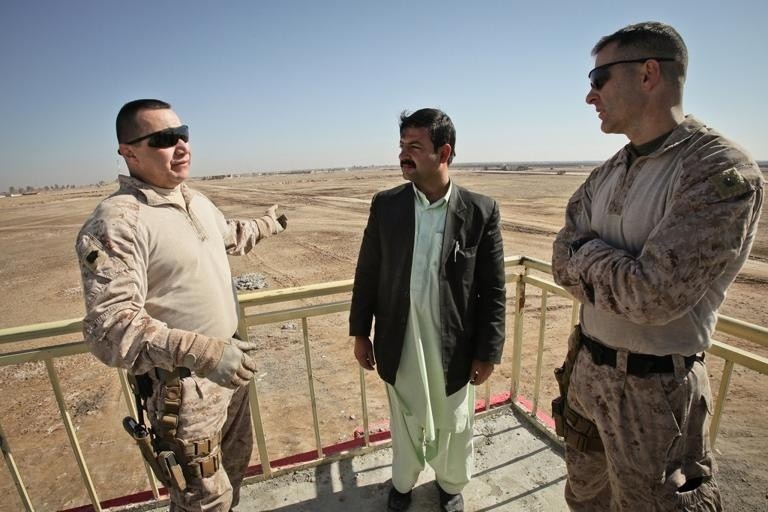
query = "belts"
[582,336,697,377]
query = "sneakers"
[435,480,463,512]
[388,486,412,511]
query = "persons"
[547,14,768,511]
[344,106,505,510]
[68,98,291,509]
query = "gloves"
[167,327,258,390]
[253,204,288,241]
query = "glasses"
[588,57,675,91]
[117,124,190,156]
